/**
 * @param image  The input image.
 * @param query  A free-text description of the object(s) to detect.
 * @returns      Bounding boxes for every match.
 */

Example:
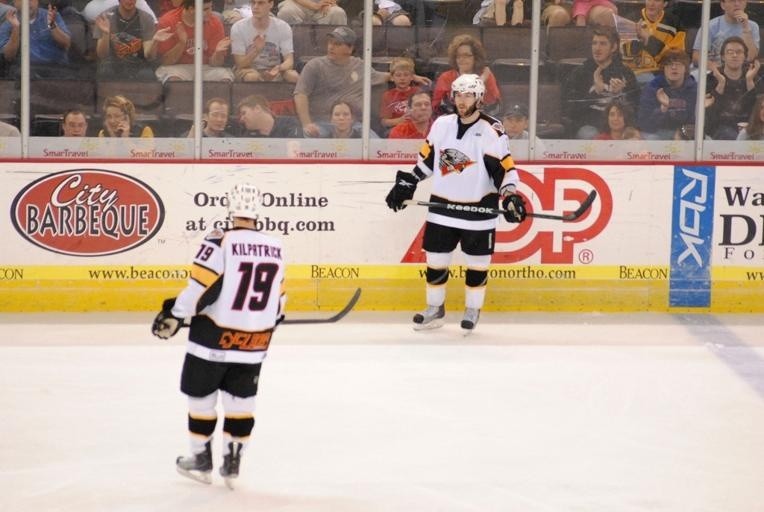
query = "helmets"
[228,182,262,219]
[451,74,485,109]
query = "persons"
[385,75,527,328]
[63,92,300,140]
[151,185,286,478]
[0,0,411,81]
[475,0,763,139]
[291,27,538,143]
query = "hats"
[327,27,356,43]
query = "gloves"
[152,297,183,339]
[386,171,419,211]
[502,195,526,222]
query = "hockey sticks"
[400,189,596,222]
[183,288,364,328]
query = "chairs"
[0,2,763,135]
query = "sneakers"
[220,442,242,476]
[413,304,444,323]
[461,307,479,329]
[177,441,212,471]
